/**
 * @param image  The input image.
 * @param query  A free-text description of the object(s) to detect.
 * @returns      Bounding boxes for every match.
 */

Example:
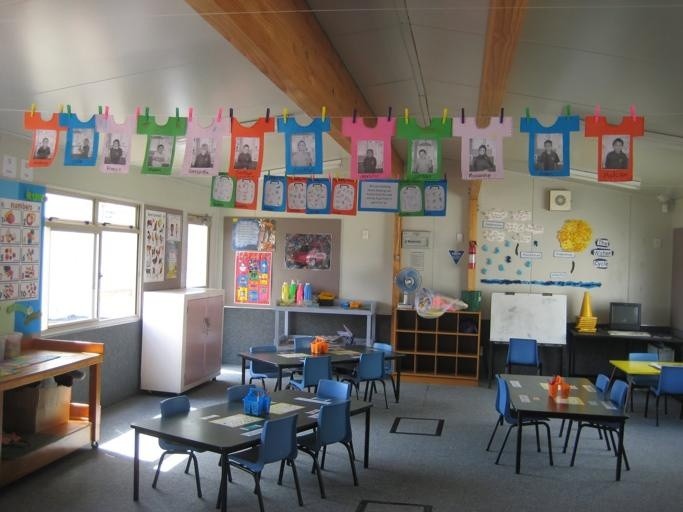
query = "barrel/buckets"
[281,279,289,306]
[288,278,297,305]
[304,282,313,306]
[295,280,304,306]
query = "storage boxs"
[3,383,71,438]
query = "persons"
[292,140,311,167]
[33,137,50,160]
[192,144,211,167]
[109,140,125,165]
[537,141,559,172]
[151,144,169,167]
[362,149,376,173]
[606,139,628,171]
[237,144,251,170]
[76,137,90,158]
[473,144,493,172]
[414,149,433,174]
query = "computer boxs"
[647,343,675,362]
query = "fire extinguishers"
[469,241,477,269]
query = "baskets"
[547,375,571,399]
[310,337,328,354]
[242,388,271,416]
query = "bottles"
[281,280,312,301]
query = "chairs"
[504,338,543,377]
[485,352,682,482]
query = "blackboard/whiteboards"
[489,292,567,348]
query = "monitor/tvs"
[609,302,642,331]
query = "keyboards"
[608,330,651,336]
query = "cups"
[561,384,569,399]
[549,398,569,411]
[0,331,24,363]
[548,381,558,398]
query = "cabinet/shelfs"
[140,287,227,396]
[391,307,481,388]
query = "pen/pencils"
[257,390,270,402]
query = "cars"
[290,243,327,267]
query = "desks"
[566,324,682,375]
[272,300,377,352]
[0,337,104,488]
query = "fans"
[393,267,422,309]
